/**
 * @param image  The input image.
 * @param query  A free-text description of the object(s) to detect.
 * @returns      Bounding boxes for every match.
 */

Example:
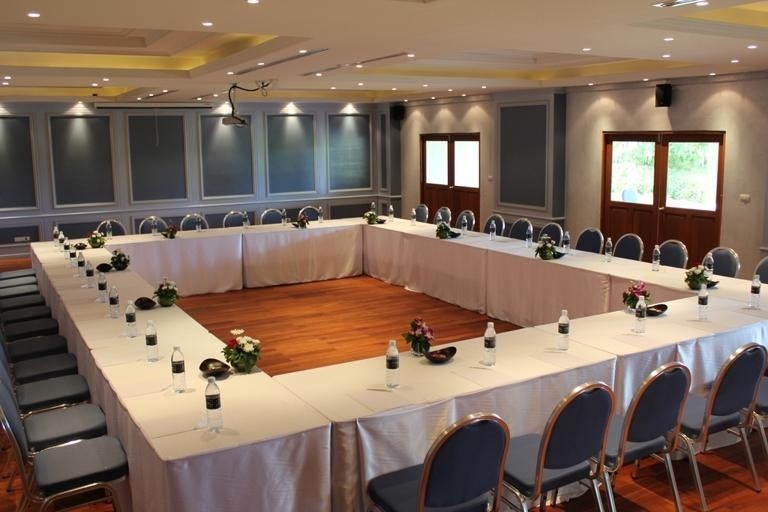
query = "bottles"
[385,338,400,389]
[51,223,224,430]
[389,205,394,220]
[489,220,497,241]
[605,237,613,262]
[750,274,762,309]
[697,283,709,321]
[558,310,570,351]
[526,224,533,248]
[635,295,648,332]
[411,208,416,225]
[483,322,497,366]
[652,245,661,271]
[436,212,442,226]
[563,232,570,254]
[104,207,325,243]
[461,216,468,235]
[704,252,715,275]
[372,203,376,214]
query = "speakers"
[654,82,671,108]
[389,103,406,121]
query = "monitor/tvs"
[222,116,248,128]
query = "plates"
[377,218,386,223]
[449,232,461,238]
[424,346,456,363]
[554,251,566,258]
[707,281,719,288]
[645,304,668,317]
